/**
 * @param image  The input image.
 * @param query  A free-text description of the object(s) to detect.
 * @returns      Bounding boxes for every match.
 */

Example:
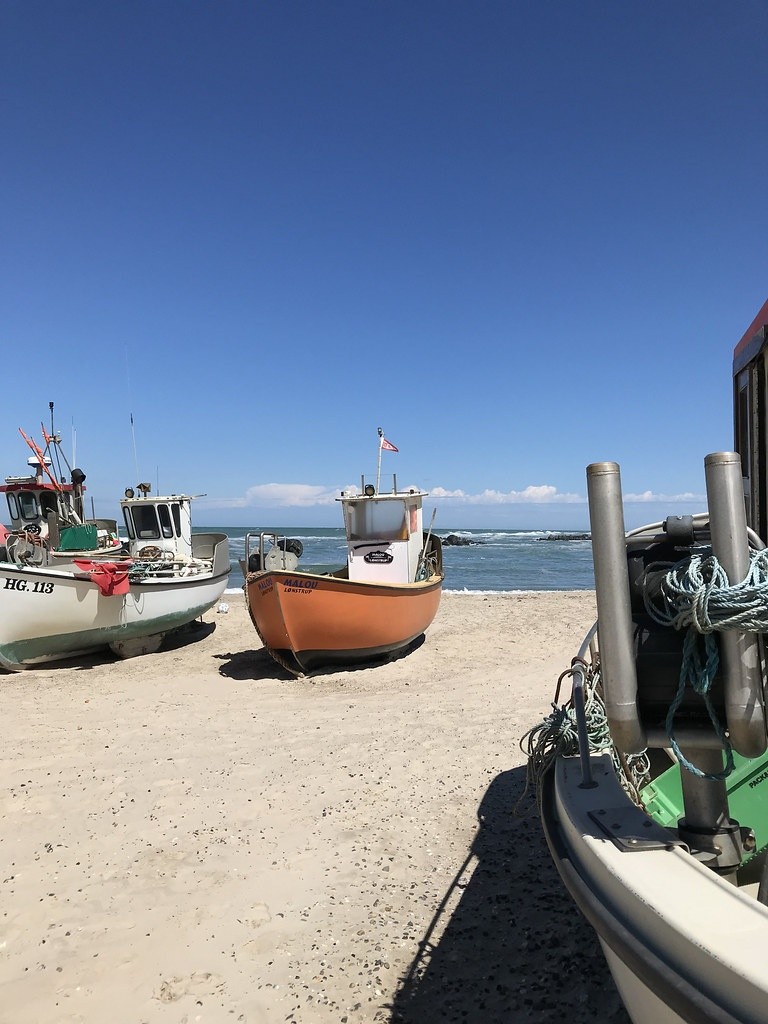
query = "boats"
[535,301,768,1023]
[237,425,446,679]
[1,400,233,674]
